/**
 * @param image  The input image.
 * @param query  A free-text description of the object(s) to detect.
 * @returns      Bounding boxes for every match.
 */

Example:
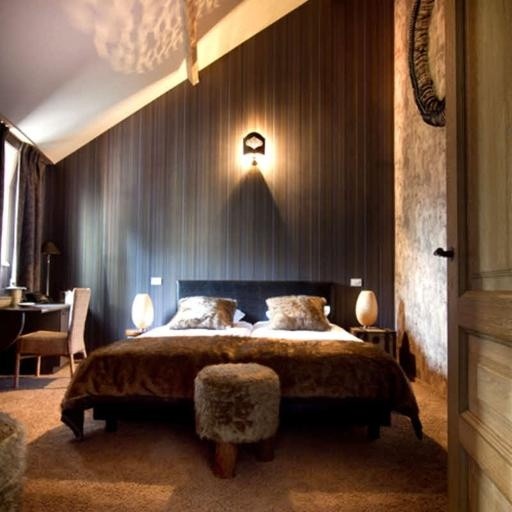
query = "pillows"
[168,296,237,329]
[265,295,332,331]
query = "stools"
[0,412,29,512]
[194,363,281,479]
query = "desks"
[0,303,70,374]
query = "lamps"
[41,242,62,298]
[356,290,378,329]
[243,132,265,166]
[131,293,154,333]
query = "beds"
[60,280,423,440]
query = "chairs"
[14,287,91,387]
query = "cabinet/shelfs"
[351,327,396,356]
[125,329,144,338]
